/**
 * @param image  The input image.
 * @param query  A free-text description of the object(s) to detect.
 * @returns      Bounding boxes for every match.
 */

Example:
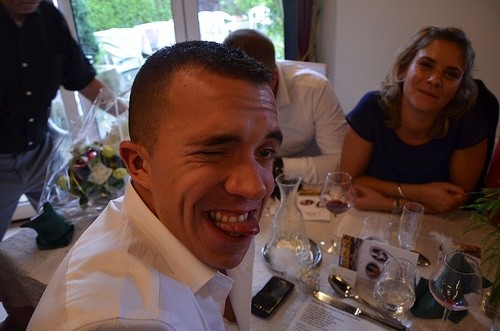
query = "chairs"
[464,77,500,211]
[294,61,326,76]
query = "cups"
[398,202,424,250]
[374,256,416,315]
[360,215,391,245]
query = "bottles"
[270,174,311,260]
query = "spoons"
[328,273,403,325]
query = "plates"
[262,235,322,273]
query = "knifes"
[312,291,410,331]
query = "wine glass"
[321,171,353,255]
[429,252,484,331]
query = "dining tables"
[0,184,500,330]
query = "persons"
[339,26,488,214]
[0,0,128,242]
[26,40,283,331]
[223,28,347,185]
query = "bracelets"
[397,182,408,199]
[393,200,400,213]
[273,159,284,181]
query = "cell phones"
[251,276,294,317]
